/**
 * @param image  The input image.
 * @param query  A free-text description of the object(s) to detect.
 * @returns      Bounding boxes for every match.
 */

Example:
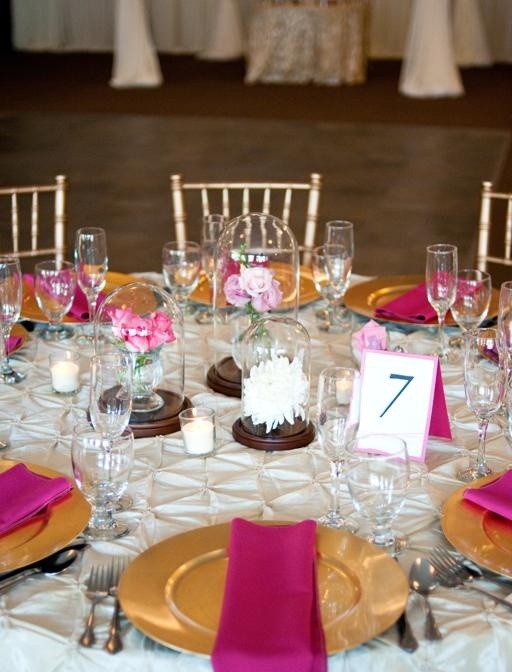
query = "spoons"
[409,557,444,643]
[0,544,79,592]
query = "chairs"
[476,181,512,287]
[169,173,323,268]
[0,175,67,276]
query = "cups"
[179,408,217,458]
[50,351,81,394]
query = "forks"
[426,546,510,610]
[77,553,131,656]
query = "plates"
[116,519,410,661]
[174,261,328,312]
[439,474,512,581]
[0,269,160,326]
[344,272,511,326]
[0,458,94,577]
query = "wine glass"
[310,219,356,333]
[316,365,408,558]
[426,242,512,481]
[163,215,226,341]
[70,355,134,540]
[0,227,108,385]
